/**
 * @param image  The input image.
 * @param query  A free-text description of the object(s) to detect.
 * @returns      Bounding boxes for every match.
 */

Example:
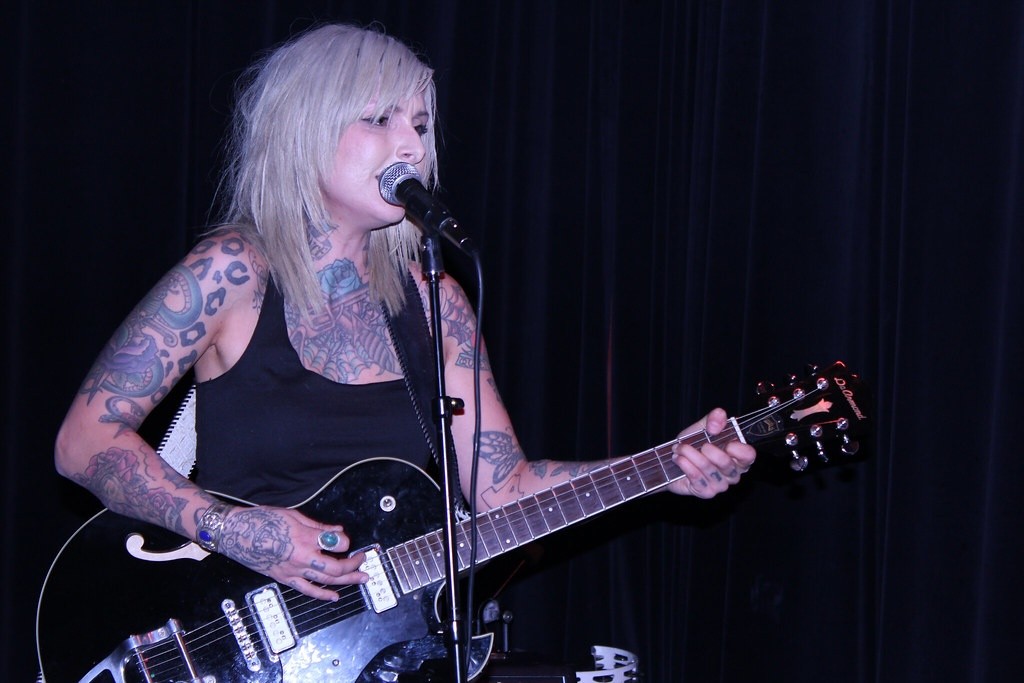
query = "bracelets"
[195,498,235,554]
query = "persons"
[53,20,756,683]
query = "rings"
[315,530,341,552]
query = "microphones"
[378,162,480,259]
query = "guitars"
[34,356,867,680]
